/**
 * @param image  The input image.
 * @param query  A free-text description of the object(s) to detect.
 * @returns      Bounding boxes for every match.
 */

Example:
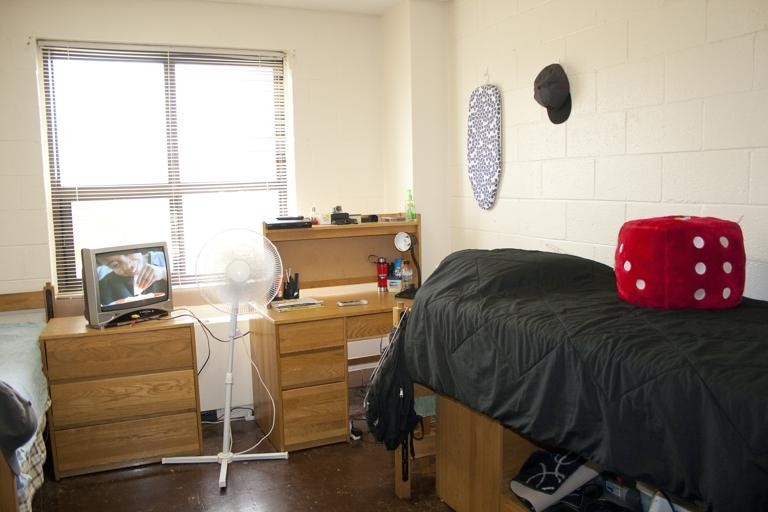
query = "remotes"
[276,215,305,220]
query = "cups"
[376,256,388,292]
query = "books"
[270,295,320,314]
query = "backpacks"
[363,311,425,482]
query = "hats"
[533,63,572,124]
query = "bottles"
[402,261,413,291]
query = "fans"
[161,227,290,489]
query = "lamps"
[393,231,421,299]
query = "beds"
[0,282,54,512]
[392,249,768,512]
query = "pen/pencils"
[285,267,292,283]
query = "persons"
[96,248,168,310]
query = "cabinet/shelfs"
[39,306,202,480]
[250,213,422,452]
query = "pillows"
[615,214,746,312]
[440,250,615,298]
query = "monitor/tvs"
[79,240,174,330]
